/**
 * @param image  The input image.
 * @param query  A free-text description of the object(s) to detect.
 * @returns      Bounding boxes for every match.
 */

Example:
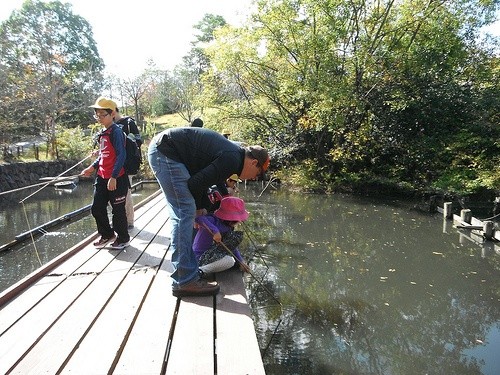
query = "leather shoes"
[173,277,220,296]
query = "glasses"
[93,113,109,119]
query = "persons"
[80,97,131,250]
[99,105,141,229]
[147,119,270,296]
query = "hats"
[230,174,242,182]
[214,197,250,221]
[246,145,270,179]
[89,96,115,110]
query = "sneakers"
[110,238,130,249]
[93,234,116,246]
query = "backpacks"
[109,125,141,175]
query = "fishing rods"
[250,269,268,302]
[242,221,263,246]
[199,220,283,307]
[19,154,92,204]
[240,222,269,269]
[247,246,262,265]
[0,174,84,195]
[262,319,282,359]
